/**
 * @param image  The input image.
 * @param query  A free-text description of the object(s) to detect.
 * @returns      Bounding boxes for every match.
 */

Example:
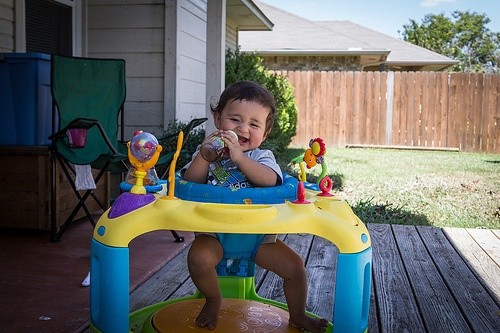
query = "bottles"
[200,130,238,162]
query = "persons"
[181,82,328,333]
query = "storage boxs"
[0,52,111,233]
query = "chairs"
[48,54,208,243]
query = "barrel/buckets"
[0,53,58,146]
